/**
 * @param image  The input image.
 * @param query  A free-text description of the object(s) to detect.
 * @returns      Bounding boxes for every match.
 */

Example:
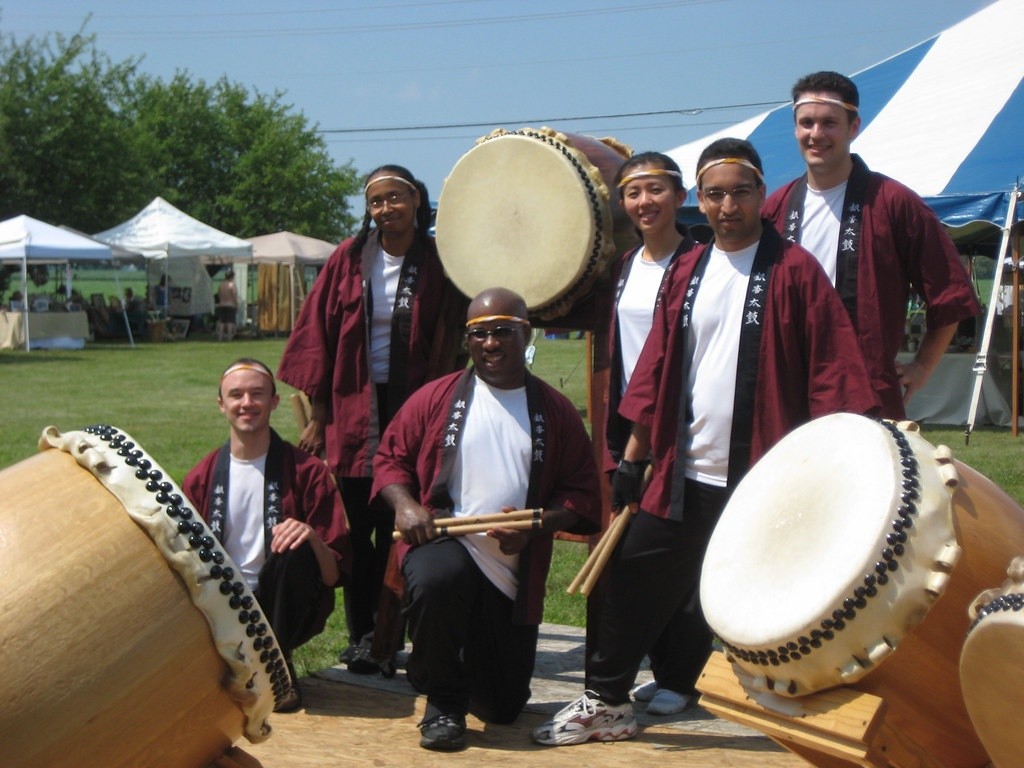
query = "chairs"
[148,309,189,340]
[90,293,111,341]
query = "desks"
[0,310,88,350]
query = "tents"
[55,195,345,342]
[0,214,138,349]
[648,0,1024,443]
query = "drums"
[698,409,1024,768]
[432,124,650,332]
[0,420,301,767]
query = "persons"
[372,289,606,751]
[179,356,352,711]
[151,274,175,343]
[272,165,476,676]
[122,288,146,338]
[523,71,983,748]
[216,268,240,343]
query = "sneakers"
[531,689,638,745]
[632,680,659,701]
[419,707,466,749]
[646,689,692,714]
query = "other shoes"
[278,662,301,709]
[347,656,378,675]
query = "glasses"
[467,325,525,341]
[699,187,757,203]
[365,191,413,213]
